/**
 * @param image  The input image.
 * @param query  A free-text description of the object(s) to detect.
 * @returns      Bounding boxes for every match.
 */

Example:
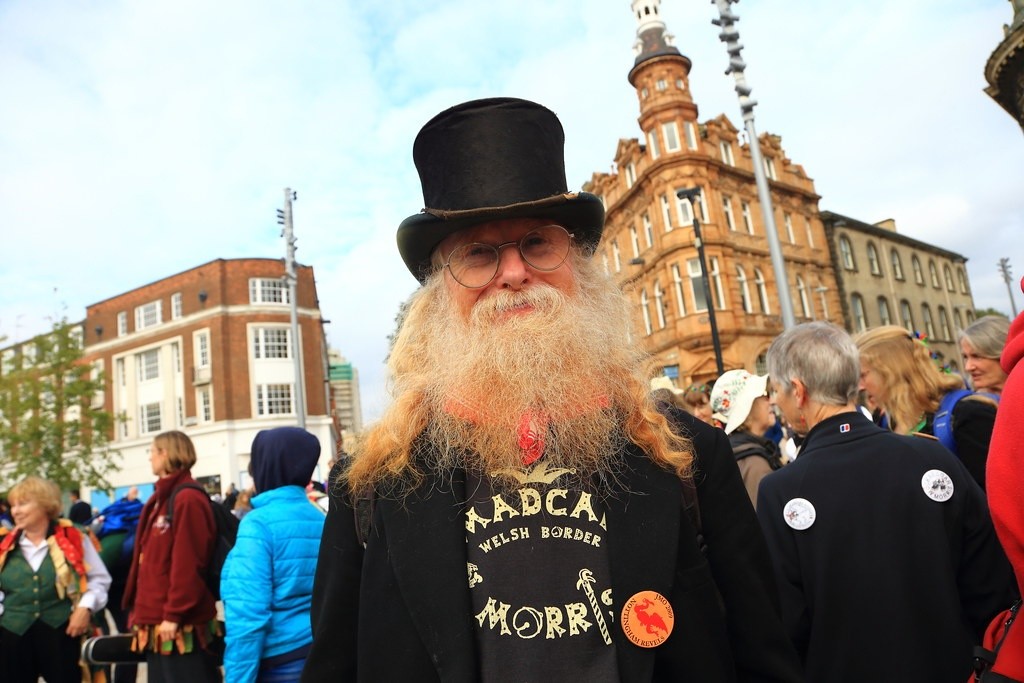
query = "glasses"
[445,224,574,289]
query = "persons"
[648,368,804,513]
[308,95,805,683]
[754,320,1014,683]
[120,430,220,682]
[0,476,114,683]
[218,427,327,683]
[968,278,1024,683]
[201,481,255,597]
[854,312,1012,496]
[0,487,92,543]
[306,459,338,511]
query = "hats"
[650,376,683,395]
[710,369,770,435]
[397,98,604,288]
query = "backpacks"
[166,482,240,602]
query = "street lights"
[675,186,727,378]
